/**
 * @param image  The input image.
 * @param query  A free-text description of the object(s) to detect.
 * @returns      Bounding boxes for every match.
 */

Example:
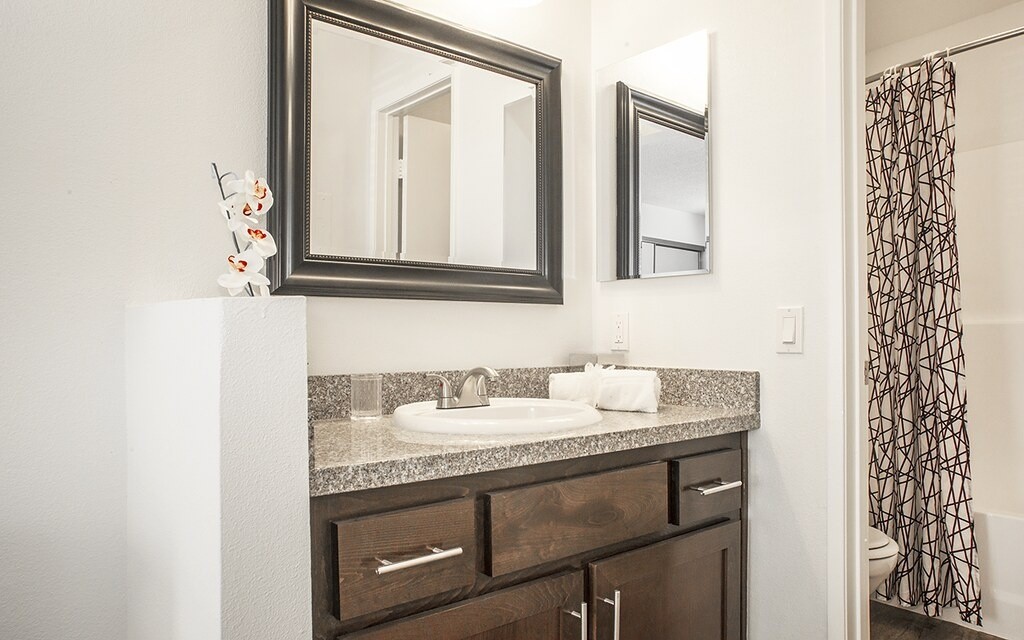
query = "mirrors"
[592,30,710,287]
[503,95,537,272]
[268,0,563,303]
[616,81,704,277]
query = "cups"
[352,375,383,419]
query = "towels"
[549,371,661,413]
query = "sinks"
[391,397,603,434]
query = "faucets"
[455,366,501,408]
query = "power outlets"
[612,313,628,350]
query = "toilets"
[868,525,899,596]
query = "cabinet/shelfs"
[311,433,749,640]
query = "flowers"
[211,163,278,295]
